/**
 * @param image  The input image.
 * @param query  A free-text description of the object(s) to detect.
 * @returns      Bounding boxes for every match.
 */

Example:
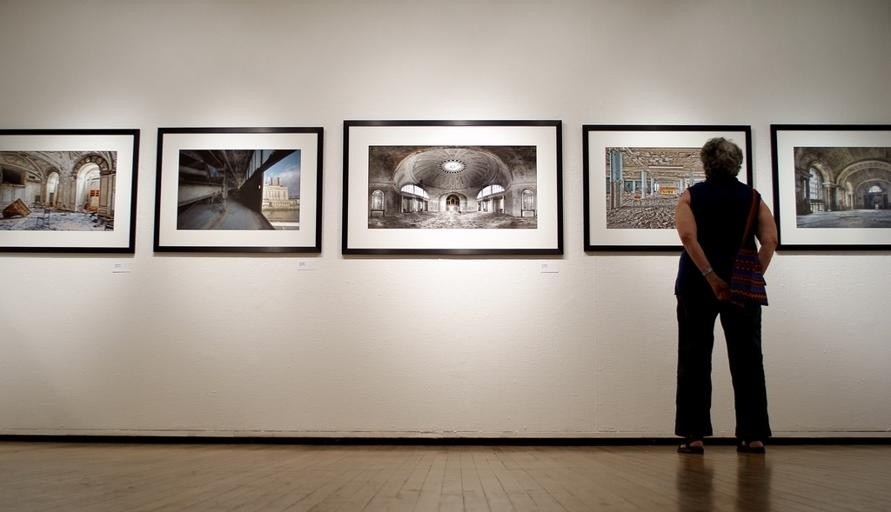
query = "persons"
[671,136,780,455]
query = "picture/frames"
[769,122,891,253]
[153,125,324,254]
[0,127,141,254]
[581,124,754,252]
[341,118,565,257]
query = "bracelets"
[699,265,712,277]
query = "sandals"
[737,439,764,453]
[677,437,704,453]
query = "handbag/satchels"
[730,189,768,308]
